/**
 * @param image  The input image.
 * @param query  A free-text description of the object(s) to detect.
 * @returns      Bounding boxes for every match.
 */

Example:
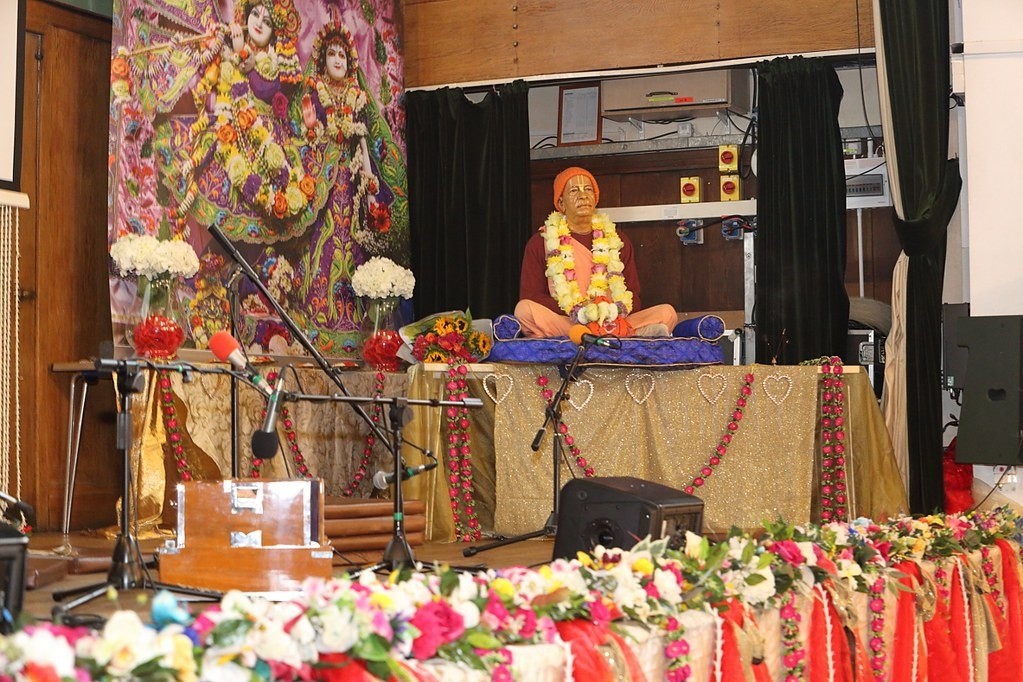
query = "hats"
[553,167,599,212]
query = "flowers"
[0,504,1023,682]
[111,234,200,279]
[351,253,416,299]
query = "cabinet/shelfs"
[601,69,750,122]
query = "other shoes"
[634,323,671,337]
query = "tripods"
[286,391,485,580]
[51,358,243,619]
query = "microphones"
[581,332,619,350]
[210,330,274,397]
[373,463,438,489]
[251,365,288,460]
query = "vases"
[359,302,403,371]
[125,274,188,361]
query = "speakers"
[954,315,1023,465]
[552,476,705,563]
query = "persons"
[514,167,679,339]
